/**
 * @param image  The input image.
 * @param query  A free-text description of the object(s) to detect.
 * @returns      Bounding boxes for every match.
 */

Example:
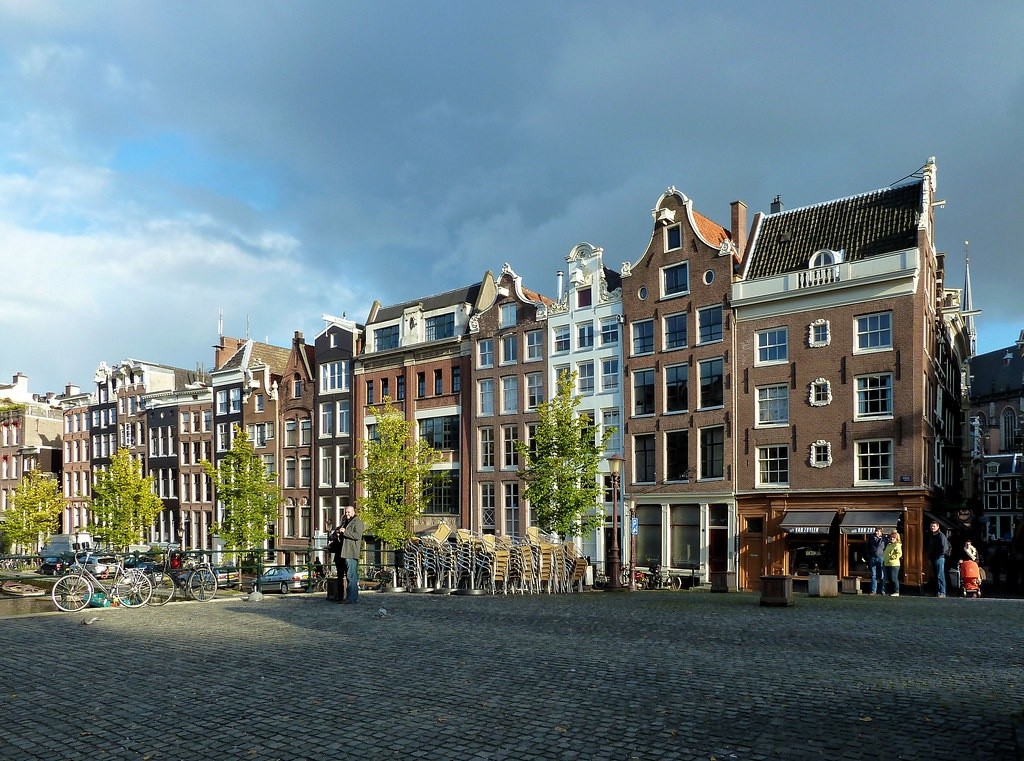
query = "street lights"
[606,452,626,589]
[326,519,333,575]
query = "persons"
[882,532,903,596]
[55,562,110,579]
[929,520,948,598]
[957,539,981,598]
[329,506,363,604]
[865,527,886,596]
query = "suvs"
[40,558,70,576]
[68,556,119,580]
[124,557,154,573]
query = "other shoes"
[938,594,946,598]
[869,592,875,595]
[338,600,348,604]
[891,593,899,596]
[881,592,885,595]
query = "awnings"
[779,512,836,534]
[839,511,901,534]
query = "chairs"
[404,524,588,598]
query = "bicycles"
[50,548,153,612]
[139,543,217,608]
[0,553,36,571]
[593,564,682,591]
[314,565,394,592]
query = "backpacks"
[939,532,952,558]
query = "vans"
[41,532,93,559]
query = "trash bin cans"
[325,577,348,600]
[709,557,729,593]
[949,568,958,587]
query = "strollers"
[958,560,982,598]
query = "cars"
[180,565,239,592]
[250,567,317,594]
